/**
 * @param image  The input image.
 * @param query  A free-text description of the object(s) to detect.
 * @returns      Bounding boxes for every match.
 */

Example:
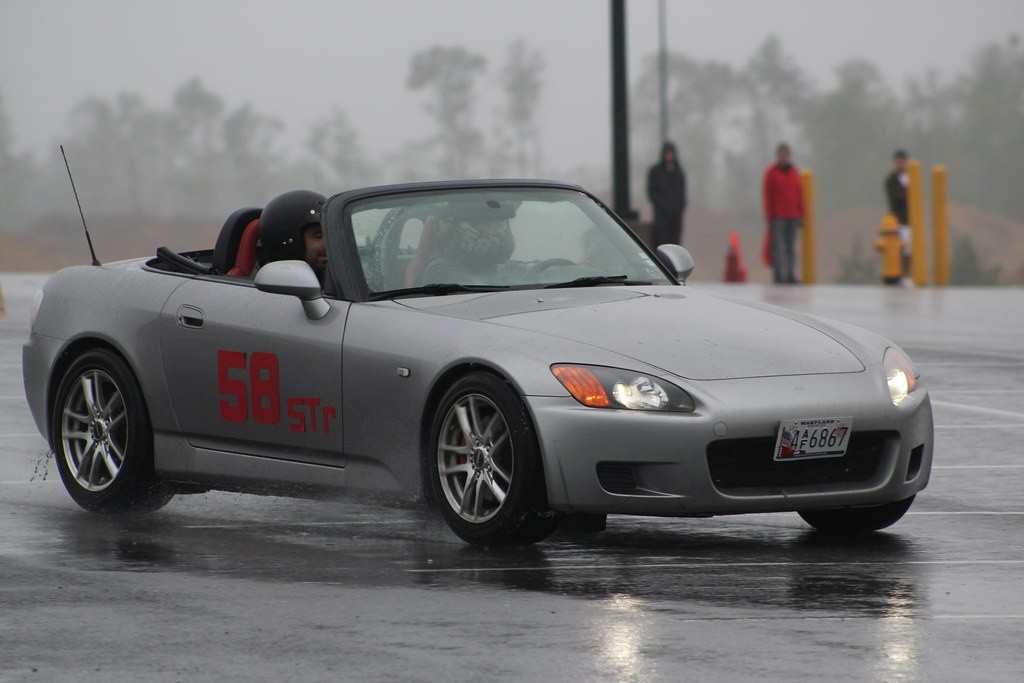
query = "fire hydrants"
[871,210,908,285]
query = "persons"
[649,143,912,285]
[249,188,377,293]
[418,203,543,289]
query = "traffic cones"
[722,231,755,284]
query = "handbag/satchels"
[760,225,773,268]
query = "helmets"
[445,210,515,263]
[258,190,329,268]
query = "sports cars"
[21,175,934,550]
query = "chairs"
[404,214,438,289]
[210,208,263,278]
